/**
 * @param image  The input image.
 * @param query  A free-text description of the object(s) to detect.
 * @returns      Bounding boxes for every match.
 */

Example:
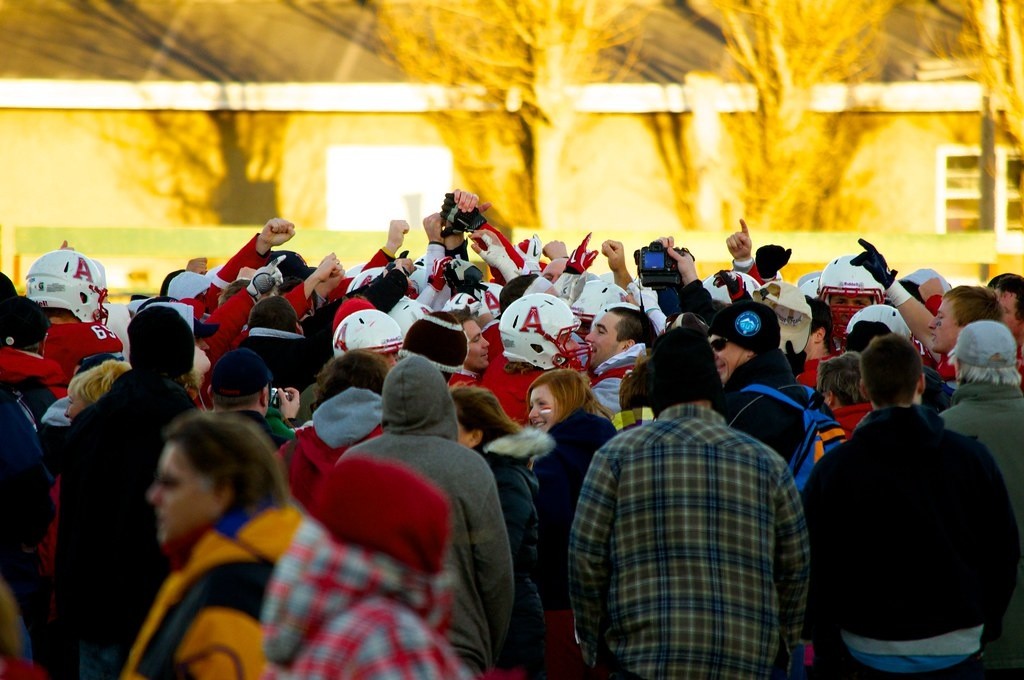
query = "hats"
[270,251,316,291]
[42,322,125,382]
[314,458,449,579]
[709,272,831,350]
[948,320,1017,367]
[128,264,226,369]
[211,296,297,397]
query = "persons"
[0,188,1024,680]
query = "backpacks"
[739,382,847,491]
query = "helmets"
[328,264,503,373]
[845,304,911,340]
[25,249,111,324]
[571,280,627,320]
[797,254,884,339]
[499,293,592,371]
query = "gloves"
[787,641,814,680]
[714,270,746,300]
[849,238,898,291]
[563,231,599,274]
[428,256,453,292]
[756,244,792,280]
[629,278,660,311]
[441,193,487,237]
[524,234,542,262]
[247,254,286,299]
[783,340,806,374]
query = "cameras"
[637,242,687,291]
[270,388,290,406]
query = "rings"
[337,261,339,265]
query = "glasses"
[711,338,728,351]
